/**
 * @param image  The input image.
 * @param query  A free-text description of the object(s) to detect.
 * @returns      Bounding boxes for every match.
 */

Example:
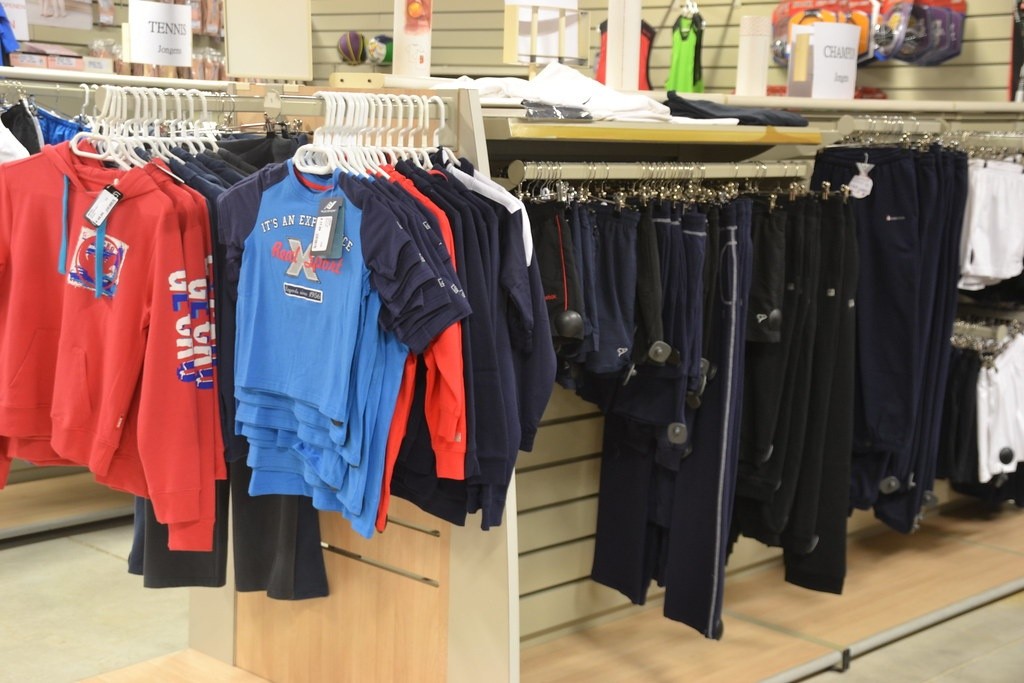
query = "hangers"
[519,111,1024,368]
[0,76,462,185]
[675,0,706,30]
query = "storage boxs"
[47,56,84,70]
[786,21,861,99]
[8,51,47,69]
[84,55,116,73]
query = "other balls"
[368,34,393,66]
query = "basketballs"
[337,31,369,66]
